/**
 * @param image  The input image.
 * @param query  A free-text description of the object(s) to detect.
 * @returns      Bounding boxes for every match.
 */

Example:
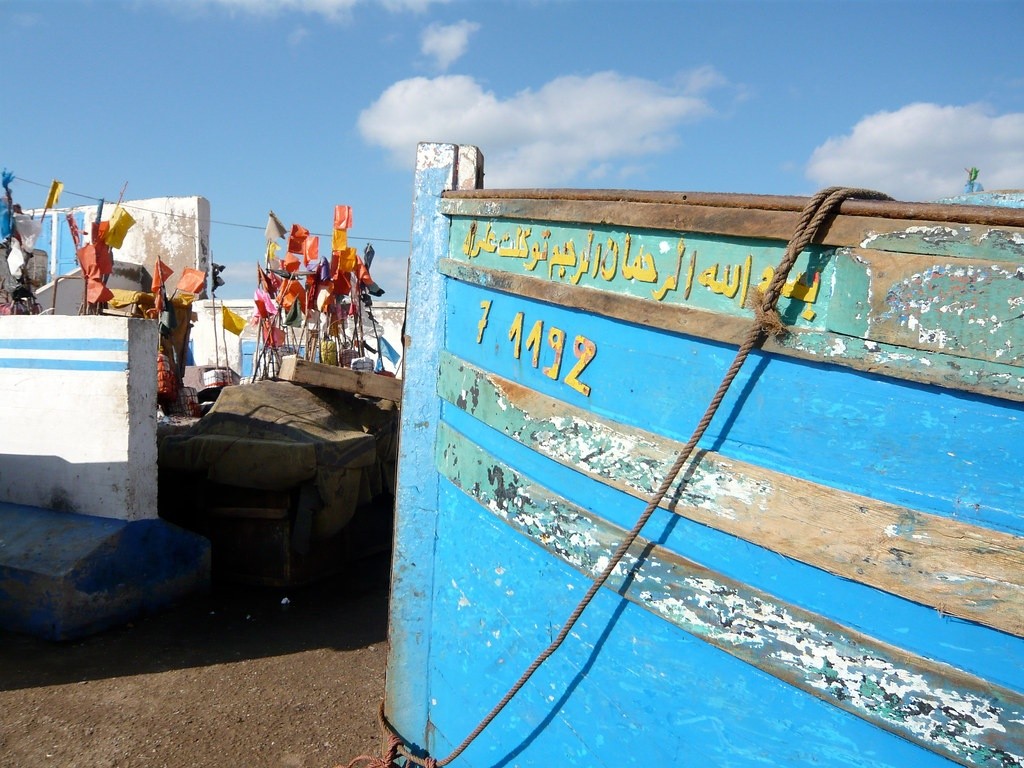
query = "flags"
[222,204,384,350]
[44,180,207,321]
